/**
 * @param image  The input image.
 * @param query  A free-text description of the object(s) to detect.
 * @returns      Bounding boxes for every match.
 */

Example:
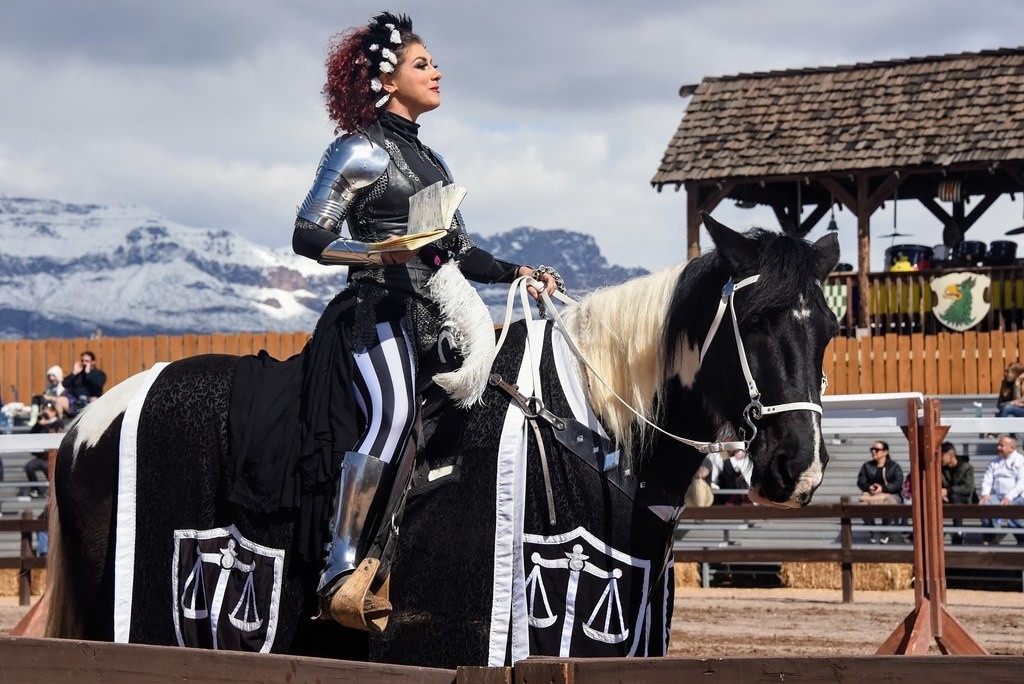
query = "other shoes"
[952,533,958,545]
[957,532,964,544]
[879,534,890,545]
[29,489,40,498]
[868,531,877,544]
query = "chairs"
[884,241,1018,272]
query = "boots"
[316,450,392,620]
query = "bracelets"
[388,251,398,265]
[517,265,523,287]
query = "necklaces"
[391,130,425,161]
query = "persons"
[23,351,108,498]
[831,247,1022,331]
[941,440,980,546]
[291,9,558,619]
[856,439,904,543]
[689,426,758,506]
[987,361,1024,441]
[979,433,1024,547]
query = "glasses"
[870,448,887,452]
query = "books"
[365,180,468,250]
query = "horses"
[39,211,842,669]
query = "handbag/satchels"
[859,492,897,505]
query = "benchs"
[673,392,1024,549]
[0,423,49,558]
